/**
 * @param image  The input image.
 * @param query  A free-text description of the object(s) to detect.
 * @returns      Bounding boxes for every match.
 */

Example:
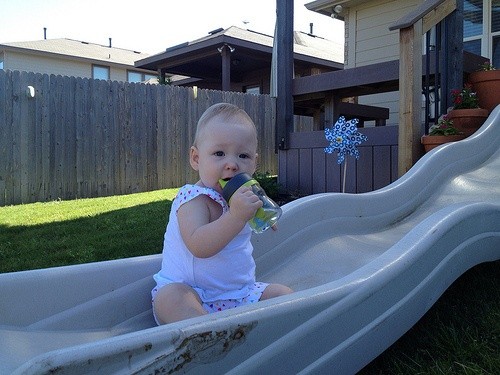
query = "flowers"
[481,61,494,70]
[451,83,480,109]
[428,114,460,136]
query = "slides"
[0,104,500,375]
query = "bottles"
[219,172,283,233]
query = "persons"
[152,103,296,325]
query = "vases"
[470,70,500,114]
[448,109,488,138]
[421,132,465,153]
